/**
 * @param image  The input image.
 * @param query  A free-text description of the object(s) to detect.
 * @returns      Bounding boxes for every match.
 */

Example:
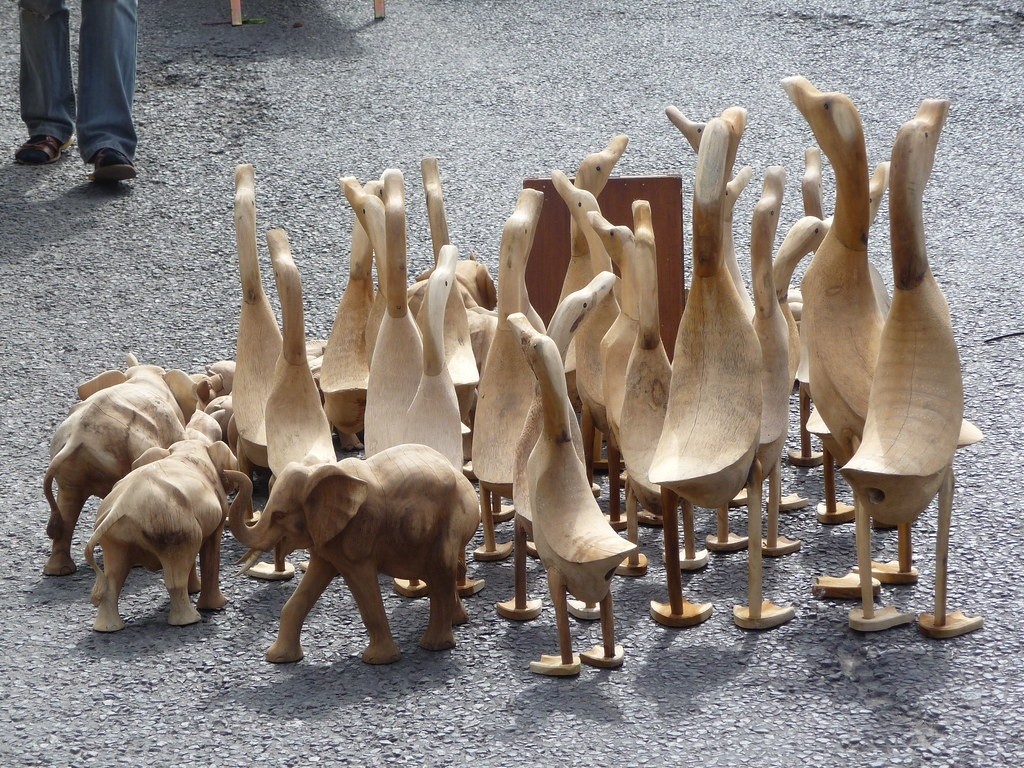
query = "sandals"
[88,146,137,182]
[15,134,74,165]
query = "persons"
[14,0,139,181]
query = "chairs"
[513,175,689,460]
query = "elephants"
[42,352,241,636]
[217,442,484,669]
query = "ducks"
[231,73,987,678]
